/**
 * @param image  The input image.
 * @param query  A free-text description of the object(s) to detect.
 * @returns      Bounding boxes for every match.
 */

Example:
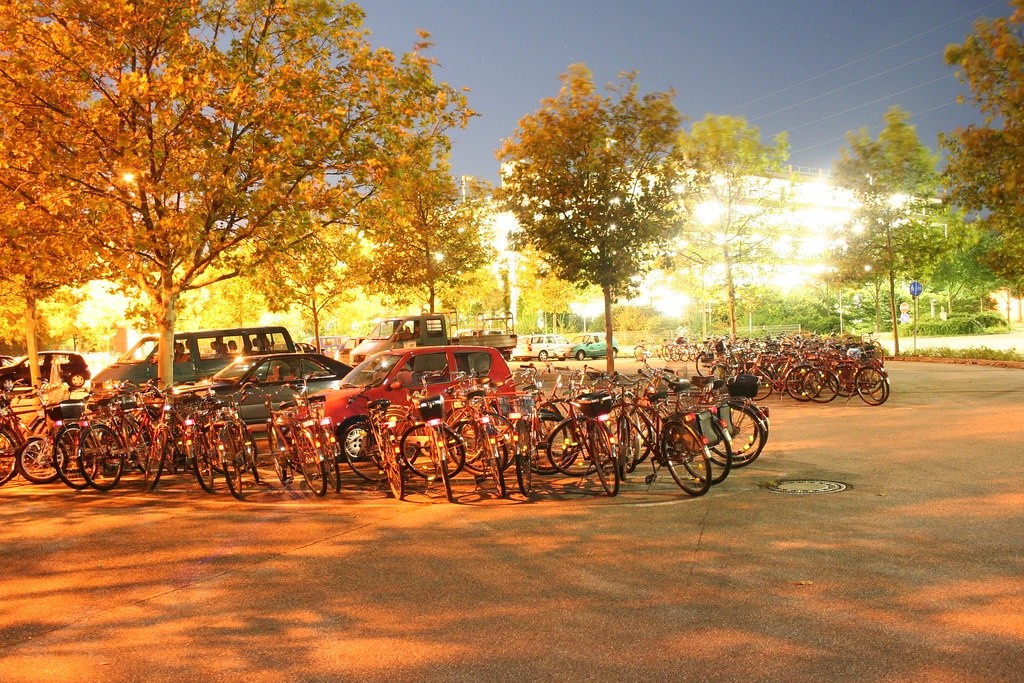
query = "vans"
[91,326,297,396]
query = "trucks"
[350,308,517,372]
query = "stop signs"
[900,302,909,313]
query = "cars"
[280,346,516,458]
[176,352,373,425]
[513,334,570,359]
[0,352,90,390]
[250,337,366,362]
[456,329,506,336]
[554,333,619,361]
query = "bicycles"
[632,330,891,406]
[0,365,771,502]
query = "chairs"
[267,366,298,381]
[174,338,272,362]
[373,356,464,386]
